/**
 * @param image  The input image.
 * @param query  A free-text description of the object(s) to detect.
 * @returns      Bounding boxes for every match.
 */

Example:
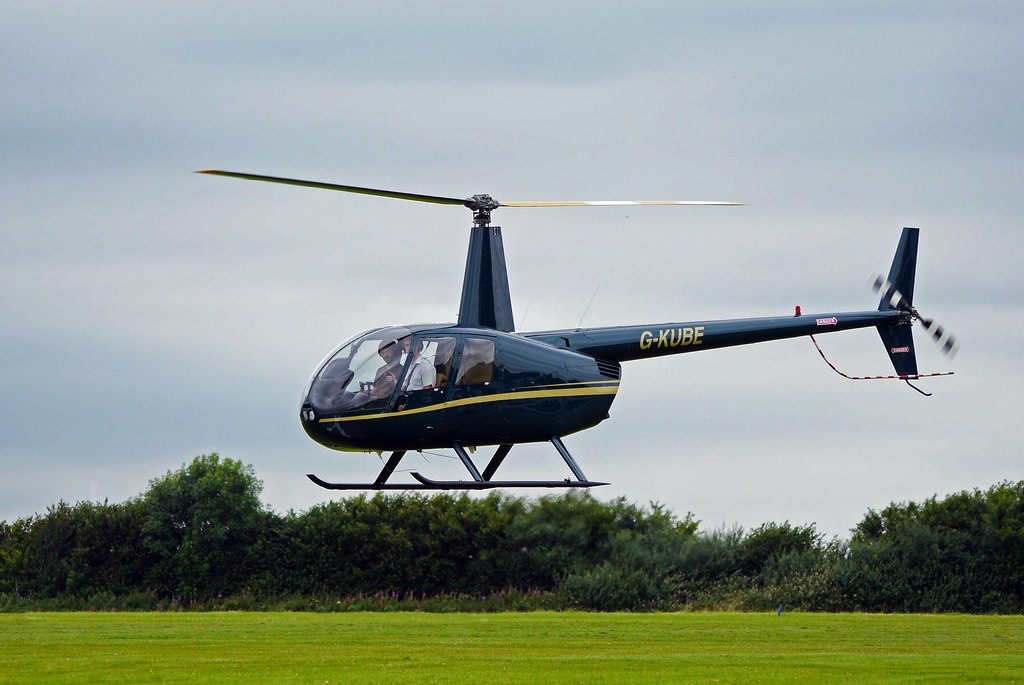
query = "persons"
[351,334,437,405]
[778,601,783,615]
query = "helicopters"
[193,166,959,492]
